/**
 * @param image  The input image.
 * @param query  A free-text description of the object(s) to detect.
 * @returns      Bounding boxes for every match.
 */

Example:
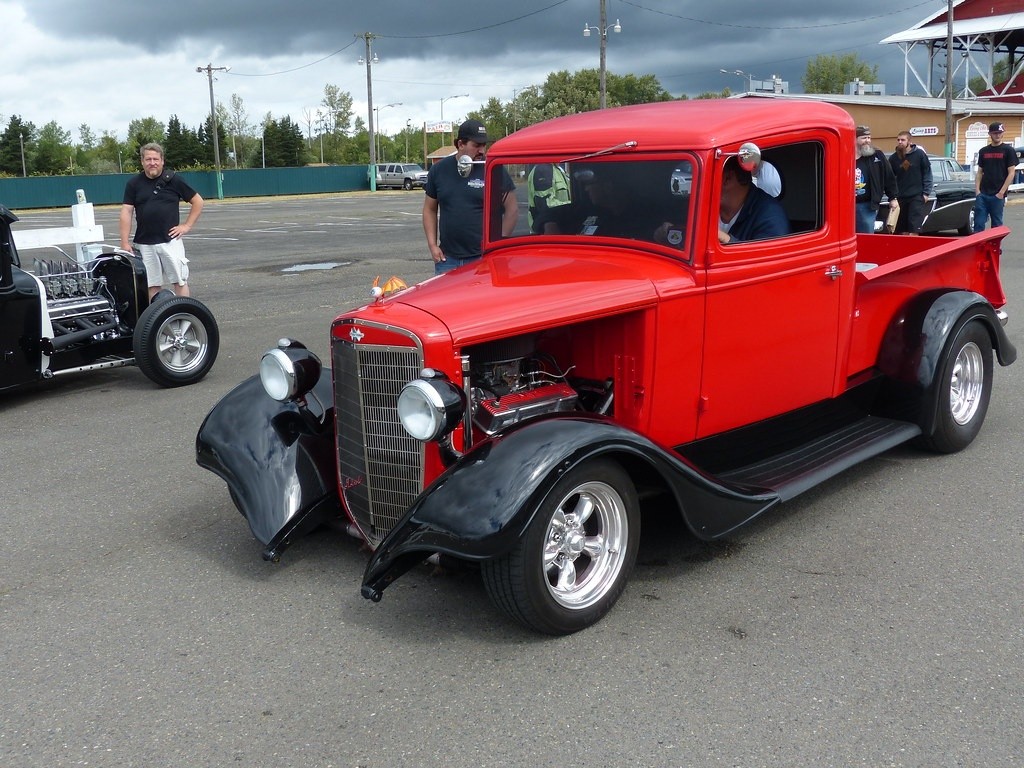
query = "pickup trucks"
[198,99,1017,639]
[367,162,430,190]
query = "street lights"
[440,94,470,147]
[356,52,376,166]
[19,133,27,177]
[584,18,621,110]
[377,102,402,165]
[119,150,123,173]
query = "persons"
[973,122,1020,235]
[888,131,933,236]
[531,164,655,242]
[528,162,571,235]
[422,119,518,275]
[119,142,204,303]
[855,126,898,234]
[653,157,788,249]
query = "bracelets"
[893,198,897,200]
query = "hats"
[458,119,496,144]
[989,122,1004,134]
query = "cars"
[0,205,220,389]
[874,152,1008,237]
